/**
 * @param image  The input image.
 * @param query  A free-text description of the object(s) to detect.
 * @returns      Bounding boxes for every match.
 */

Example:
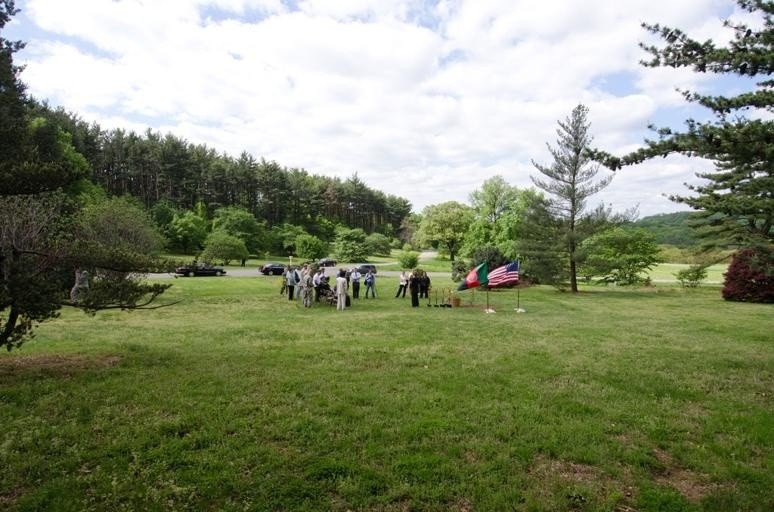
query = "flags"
[457,262,489,292]
[486,261,518,286]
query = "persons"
[278,262,431,311]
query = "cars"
[317,258,337,267]
[257,262,296,276]
[174,263,226,277]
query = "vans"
[346,264,376,275]
